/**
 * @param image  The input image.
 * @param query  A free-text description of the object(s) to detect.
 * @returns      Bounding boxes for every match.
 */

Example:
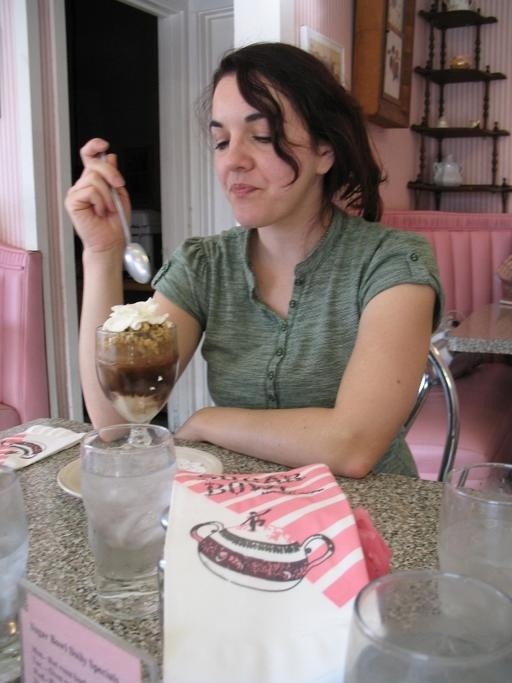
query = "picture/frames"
[299,24,346,88]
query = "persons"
[64,40,447,481]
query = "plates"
[55,442,225,501]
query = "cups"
[428,455,512,625]
[79,420,180,622]
[0,462,29,682]
[343,564,512,683]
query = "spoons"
[100,145,154,285]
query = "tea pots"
[431,151,467,187]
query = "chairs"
[368,210,511,490]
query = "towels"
[163,465,387,682]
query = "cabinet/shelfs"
[409,7,512,196]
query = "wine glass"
[92,318,181,450]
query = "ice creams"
[95,296,179,423]
